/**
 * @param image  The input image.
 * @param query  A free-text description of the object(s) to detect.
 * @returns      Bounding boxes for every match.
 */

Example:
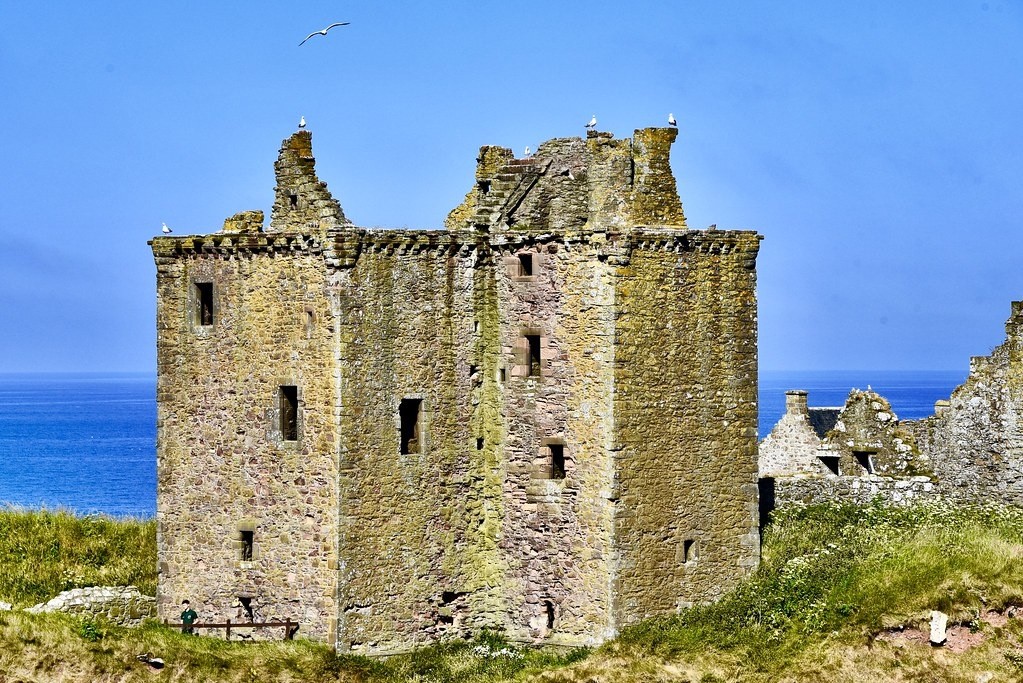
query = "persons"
[180,600,199,635]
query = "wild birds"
[523,146,531,159]
[161,223,173,236]
[298,23,351,47]
[297,116,307,131]
[667,114,678,128]
[583,115,597,132]
[850,385,872,394]
